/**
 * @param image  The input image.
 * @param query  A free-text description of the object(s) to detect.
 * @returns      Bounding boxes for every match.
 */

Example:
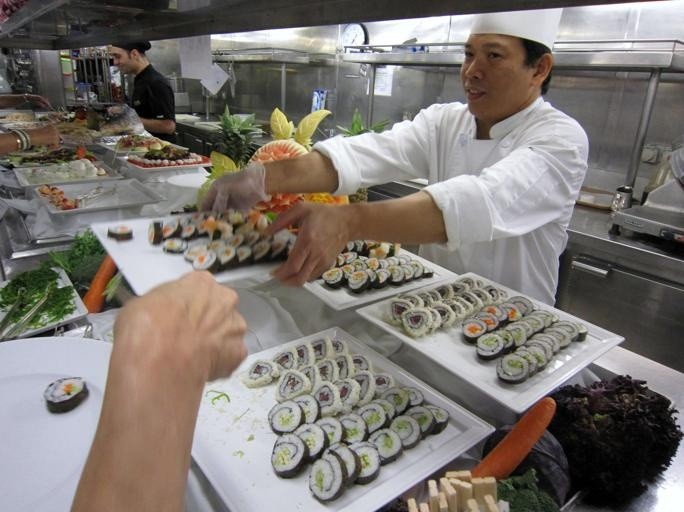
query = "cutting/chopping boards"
[195,121,223,134]
[175,114,201,123]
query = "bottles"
[611,186,633,212]
[310,88,326,115]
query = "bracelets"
[24,93,30,102]
[9,128,32,152]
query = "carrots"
[82,253,114,310]
[471,395,556,480]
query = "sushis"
[385,277,587,384]
[108,225,134,240]
[317,239,434,293]
[45,376,89,413]
[241,337,450,502]
[147,208,296,274]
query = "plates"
[2,335,113,509]
[298,237,460,314]
[188,325,496,512]
[12,134,216,217]
[354,269,627,414]
[0,267,89,339]
[88,215,300,300]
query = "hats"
[111,40,151,53]
[469,7,564,52]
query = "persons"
[71,269,246,511]
[0,93,62,156]
[197,10,590,307]
[108,39,177,144]
